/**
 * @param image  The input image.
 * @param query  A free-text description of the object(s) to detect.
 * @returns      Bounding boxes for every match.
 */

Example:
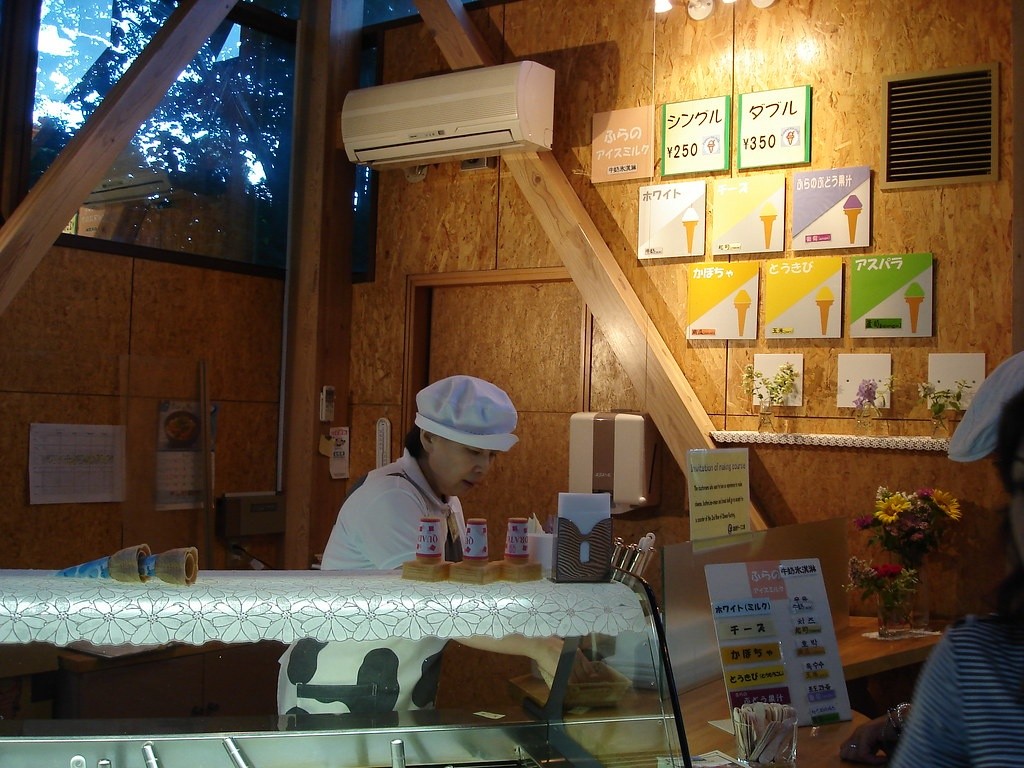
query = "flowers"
[741,361,799,433]
[854,485,963,578]
[846,555,919,631]
[850,375,896,424]
[917,378,972,433]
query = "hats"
[948,350,1024,462]
[414,375,520,452]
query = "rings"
[850,744,858,748]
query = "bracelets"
[896,702,911,726]
[888,706,900,737]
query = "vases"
[908,576,930,628]
[758,400,776,437]
[853,414,879,439]
[930,417,950,440]
[876,600,913,637]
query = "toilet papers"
[525,532,554,579]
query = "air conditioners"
[338,60,557,171]
[80,154,173,208]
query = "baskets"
[539,661,632,707]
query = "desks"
[503,617,944,768]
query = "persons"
[273,373,644,733]
[838,702,911,765]
[888,354,1024,768]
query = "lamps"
[653,0,672,14]
